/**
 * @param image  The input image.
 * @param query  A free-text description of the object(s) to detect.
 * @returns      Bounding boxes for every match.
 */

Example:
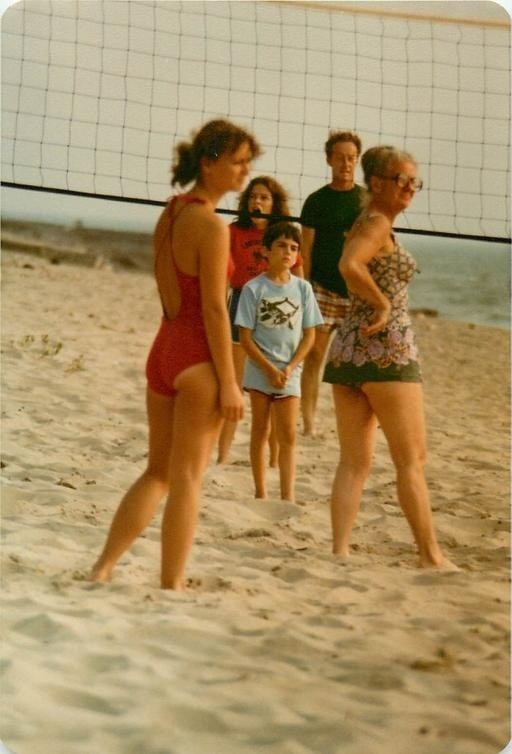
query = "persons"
[314,146,462,571]
[294,130,369,436]
[85,119,261,590]
[220,178,304,468]
[232,222,325,503]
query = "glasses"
[374,172,426,194]
